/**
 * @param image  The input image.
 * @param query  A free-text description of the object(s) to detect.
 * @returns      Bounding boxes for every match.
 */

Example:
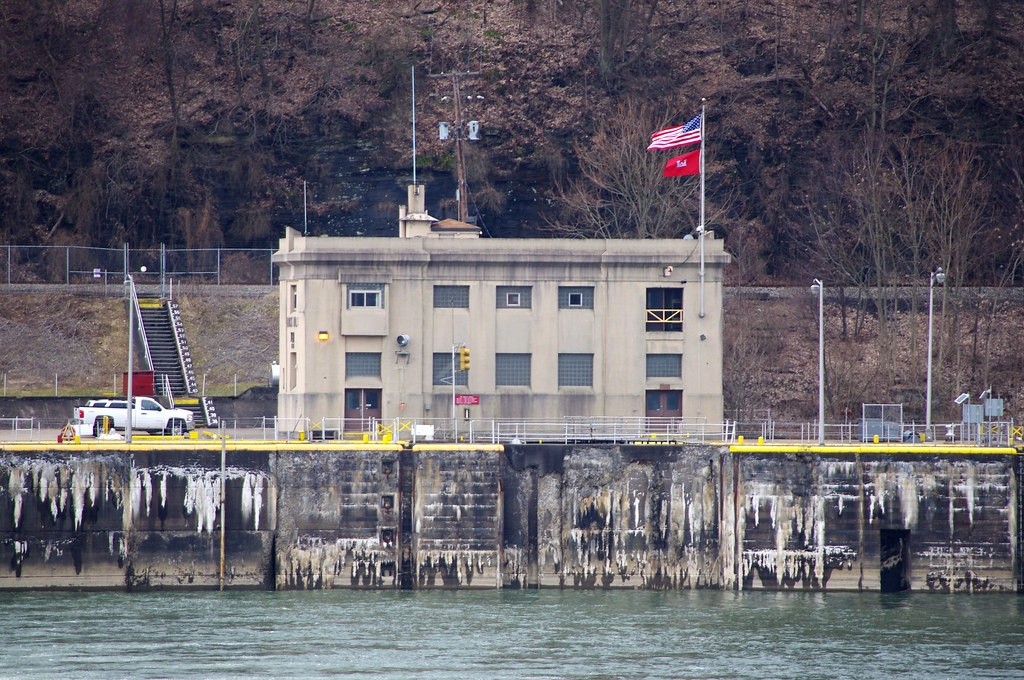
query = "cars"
[85,398,146,410]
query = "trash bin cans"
[883,422,900,441]
[857,417,883,442]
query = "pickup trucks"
[73,395,193,437]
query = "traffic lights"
[460,348,470,370]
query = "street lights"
[120,272,135,444]
[809,278,823,448]
[925,267,945,442]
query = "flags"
[646,113,702,151]
[663,148,701,177]
